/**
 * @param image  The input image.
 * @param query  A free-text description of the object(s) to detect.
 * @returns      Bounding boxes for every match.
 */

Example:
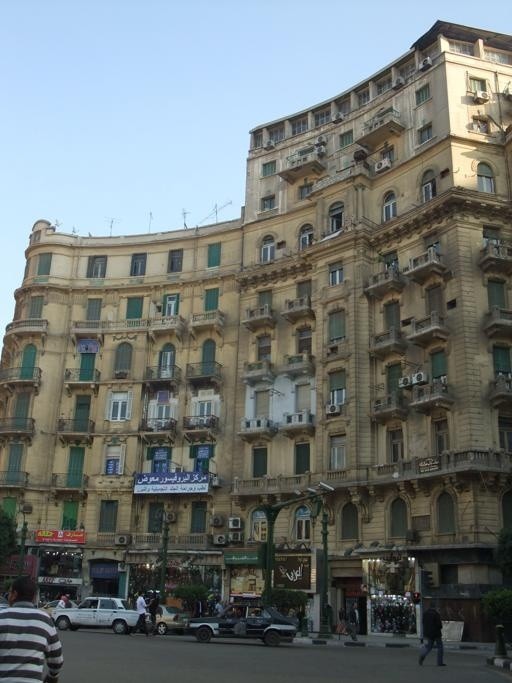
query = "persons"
[348,601,361,642]
[0,576,65,683]
[417,602,448,667]
[41,589,243,638]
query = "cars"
[39,596,79,614]
[144,604,192,635]
[188,602,301,647]
[49,594,151,633]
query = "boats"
[411,590,421,604]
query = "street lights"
[310,491,337,636]
[152,506,170,603]
[7,507,32,578]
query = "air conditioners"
[324,403,342,415]
[204,510,245,546]
[262,56,490,171]
[397,374,413,388]
[113,533,133,547]
[161,510,177,524]
